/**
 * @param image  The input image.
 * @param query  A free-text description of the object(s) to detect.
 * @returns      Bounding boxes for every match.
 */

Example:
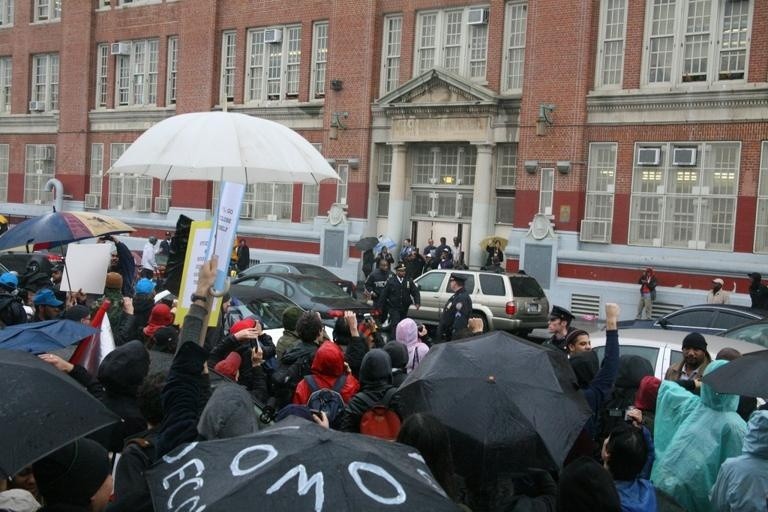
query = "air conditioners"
[39,147,52,160]
[136,198,150,213]
[673,148,696,166]
[155,198,170,213]
[466,9,489,25]
[85,194,100,209]
[111,43,127,54]
[264,29,283,43]
[239,203,252,218]
[637,148,660,166]
[29,102,44,111]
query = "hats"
[547,305,575,320]
[32,437,109,504]
[394,264,407,271]
[681,332,708,352]
[450,273,467,281]
[634,375,661,411]
[712,278,724,286]
[97,340,150,396]
[0,264,178,350]
[197,384,257,436]
[747,272,761,280]
[230,318,254,335]
[214,352,241,380]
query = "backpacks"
[355,388,403,442]
[303,374,347,424]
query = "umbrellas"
[0,205,137,290]
[100,91,344,255]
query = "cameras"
[607,405,636,422]
[251,338,259,353]
[310,409,322,422]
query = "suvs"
[366,268,550,339]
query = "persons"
[1,236,179,317]
[636,268,656,321]
[236,238,249,272]
[747,272,768,309]
[352,235,504,342]
[706,278,729,305]
[0,305,768,512]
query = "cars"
[229,272,382,331]
[585,329,767,383]
[222,284,337,358]
[715,320,768,346]
[234,262,358,300]
[0,250,64,276]
[600,305,768,337]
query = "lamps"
[327,160,336,170]
[524,161,538,173]
[536,105,553,136]
[557,161,569,174]
[329,112,346,139]
[349,159,359,169]
